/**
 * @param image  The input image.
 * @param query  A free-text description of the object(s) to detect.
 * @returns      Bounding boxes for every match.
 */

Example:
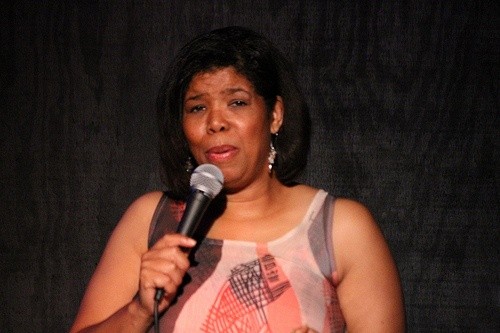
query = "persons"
[72,26,409,333]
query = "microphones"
[153,163,224,302]
